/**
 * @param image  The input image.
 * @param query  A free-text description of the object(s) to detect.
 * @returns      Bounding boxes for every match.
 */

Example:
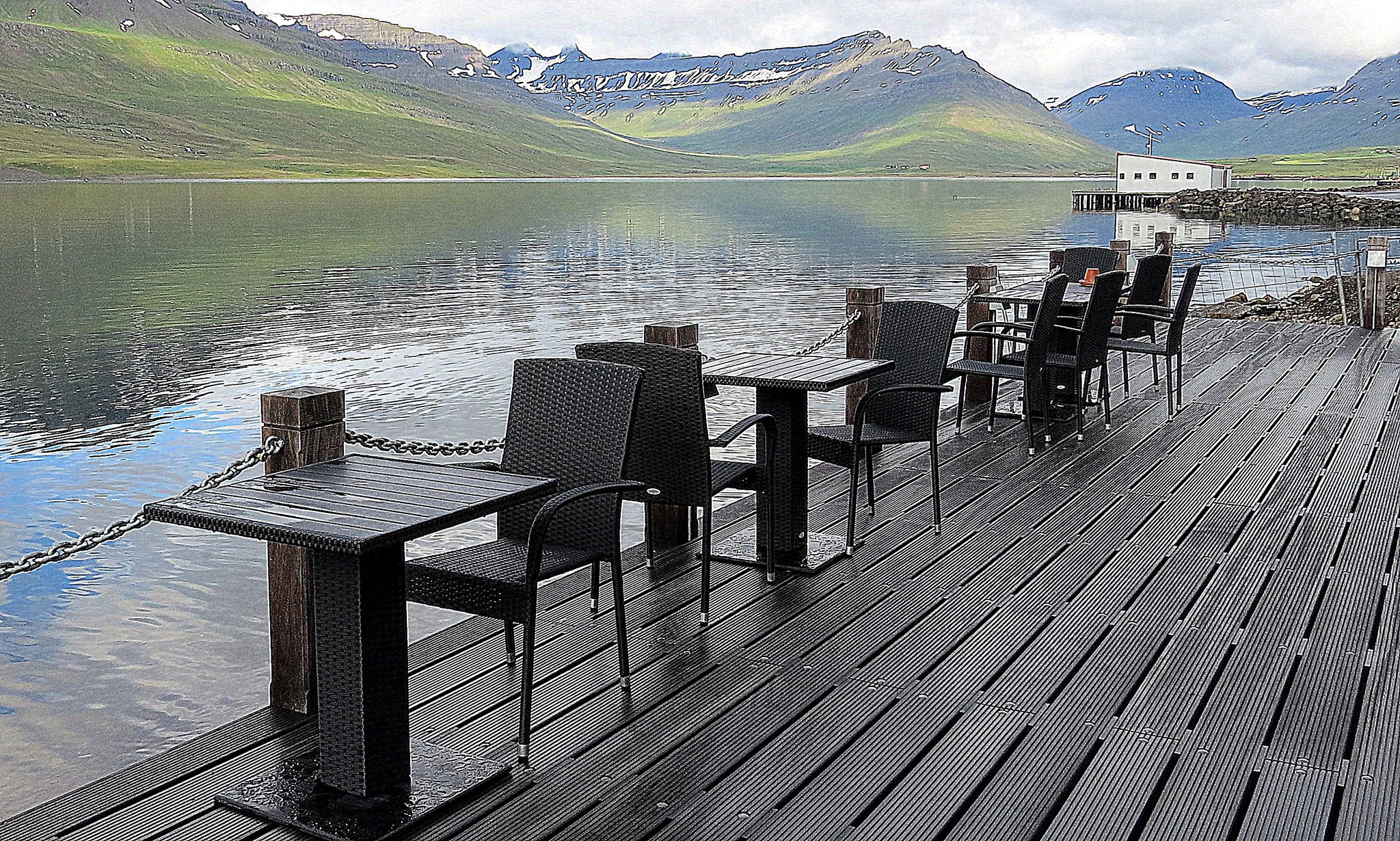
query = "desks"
[973,290,1092,433]
[144,453,558,841]
[700,351,896,571]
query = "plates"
[1079,280,1096,284]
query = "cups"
[1085,268,1099,282]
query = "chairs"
[1082,254,1172,397]
[804,300,960,557]
[988,269,1126,441]
[574,340,778,623]
[943,273,1070,455]
[1080,262,1201,421]
[405,357,647,757]
[1059,247,1119,413]
